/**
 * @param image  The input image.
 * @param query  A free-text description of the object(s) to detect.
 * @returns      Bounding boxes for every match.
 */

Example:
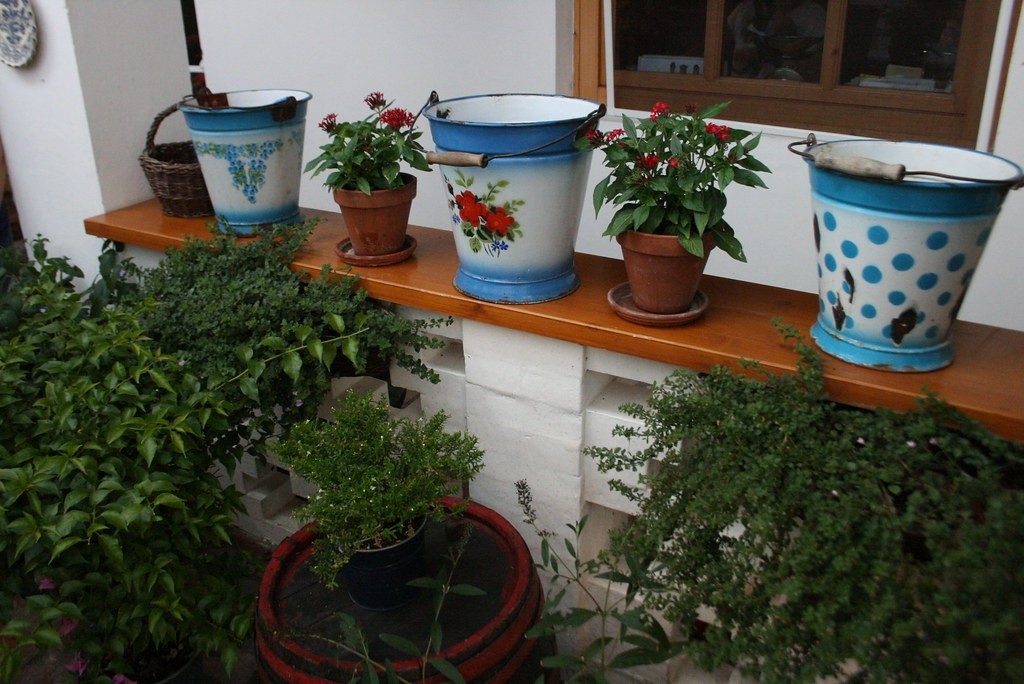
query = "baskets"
[137,100,215,218]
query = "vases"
[607,229,708,324]
[333,172,417,266]
[333,511,427,612]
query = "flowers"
[302,91,433,197]
[267,387,483,586]
[575,100,774,260]
